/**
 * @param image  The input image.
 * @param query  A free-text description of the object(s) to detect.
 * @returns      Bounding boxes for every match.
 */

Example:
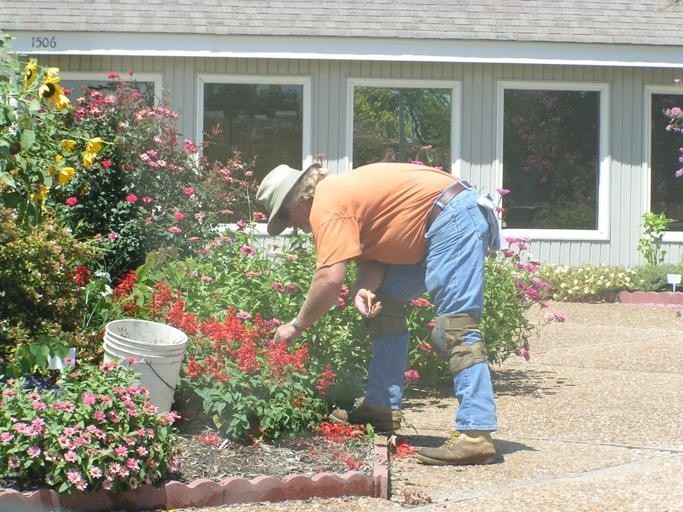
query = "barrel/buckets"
[103,317,188,415]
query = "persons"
[250,162,502,465]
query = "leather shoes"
[413,429,498,467]
[327,400,404,433]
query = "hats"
[248,159,325,238]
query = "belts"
[421,180,474,233]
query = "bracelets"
[289,320,303,332]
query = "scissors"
[366,289,374,320]
